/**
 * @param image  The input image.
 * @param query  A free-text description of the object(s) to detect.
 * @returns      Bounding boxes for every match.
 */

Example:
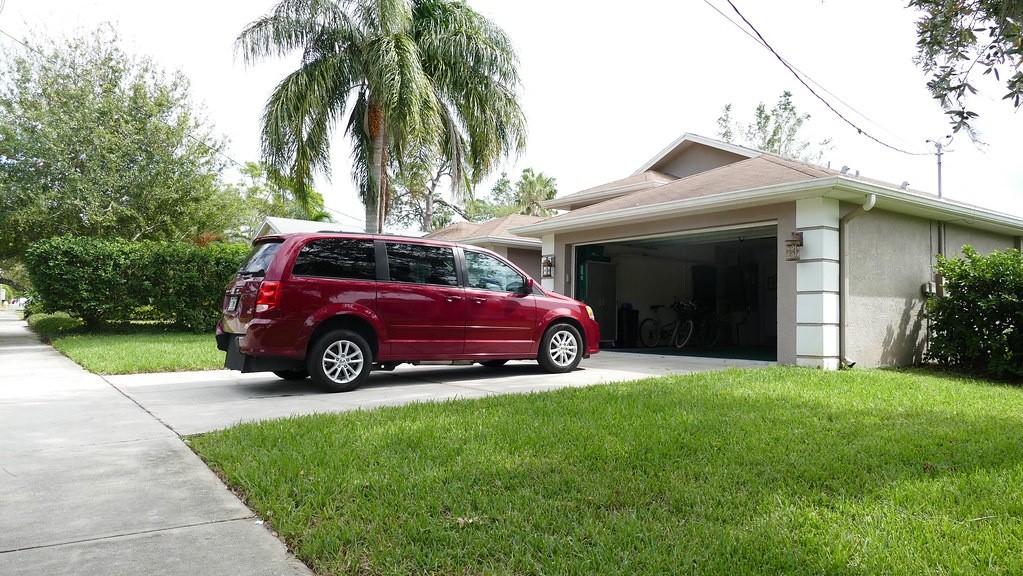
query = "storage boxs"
[581,245,604,261]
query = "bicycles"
[637,298,723,351]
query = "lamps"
[542,257,553,277]
[785,231,803,261]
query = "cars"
[216,234,601,392]
